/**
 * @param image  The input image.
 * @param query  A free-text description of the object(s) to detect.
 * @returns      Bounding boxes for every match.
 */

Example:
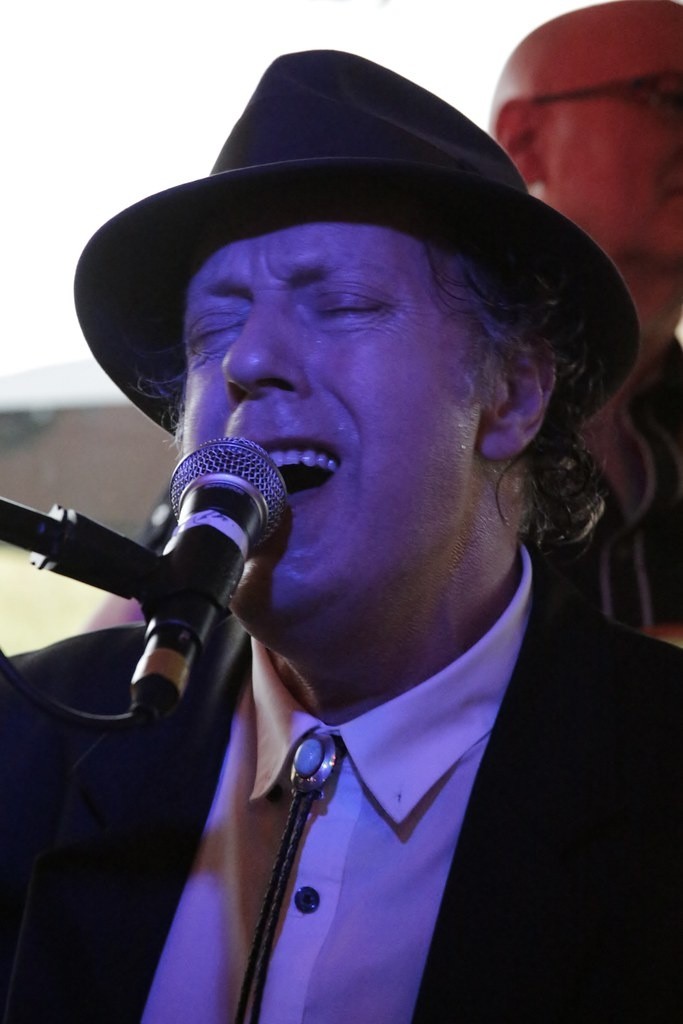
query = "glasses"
[529,70,683,129]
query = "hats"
[72,50,641,436]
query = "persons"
[2,0,683,1024]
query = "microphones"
[128,437,289,725]
